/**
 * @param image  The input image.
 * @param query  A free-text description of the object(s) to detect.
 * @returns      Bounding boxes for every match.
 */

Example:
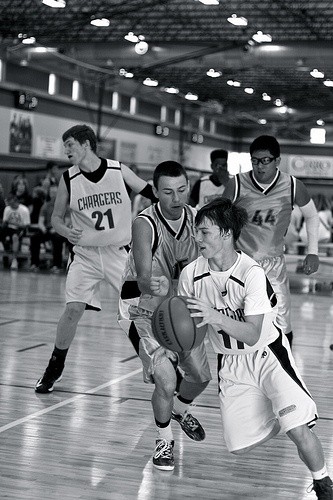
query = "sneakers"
[153,437,175,471]
[171,410,206,441]
[35,357,65,394]
[306,476,333,500]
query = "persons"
[117,160,212,473]
[222,134,321,354]
[283,202,302,243]
[32,124,159,395]
[0,160,67,274]
[299,193,333,243]
[188,150,231,213]
[178,198,333,499]
[10,113,32,155]
[122,163,159,220]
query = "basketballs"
[151,296,208,352]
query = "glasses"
[251,155,280,165]
